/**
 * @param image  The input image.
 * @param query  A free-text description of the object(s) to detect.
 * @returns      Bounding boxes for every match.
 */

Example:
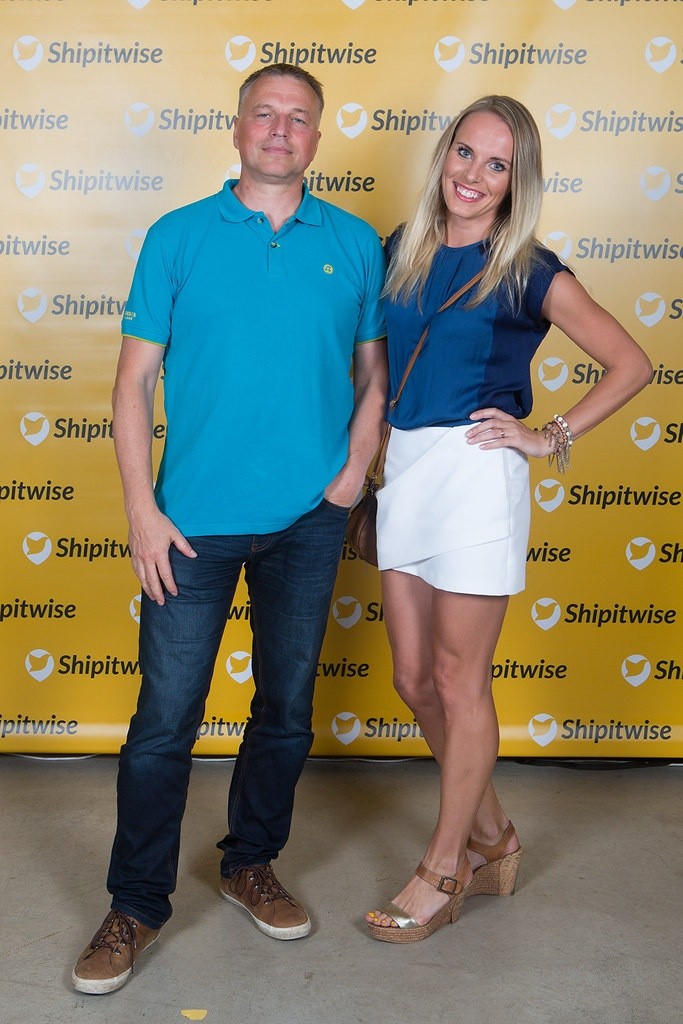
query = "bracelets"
[542,414,574,475]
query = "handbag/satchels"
[344,488,379,567]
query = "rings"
[501,428,504,439]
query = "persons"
[70,63,390,994]
[364,93,654,942]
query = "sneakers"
[219,861,312,940]
[72,909,160,994]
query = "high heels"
[367,852,474,944]
[466,820,522,897]
